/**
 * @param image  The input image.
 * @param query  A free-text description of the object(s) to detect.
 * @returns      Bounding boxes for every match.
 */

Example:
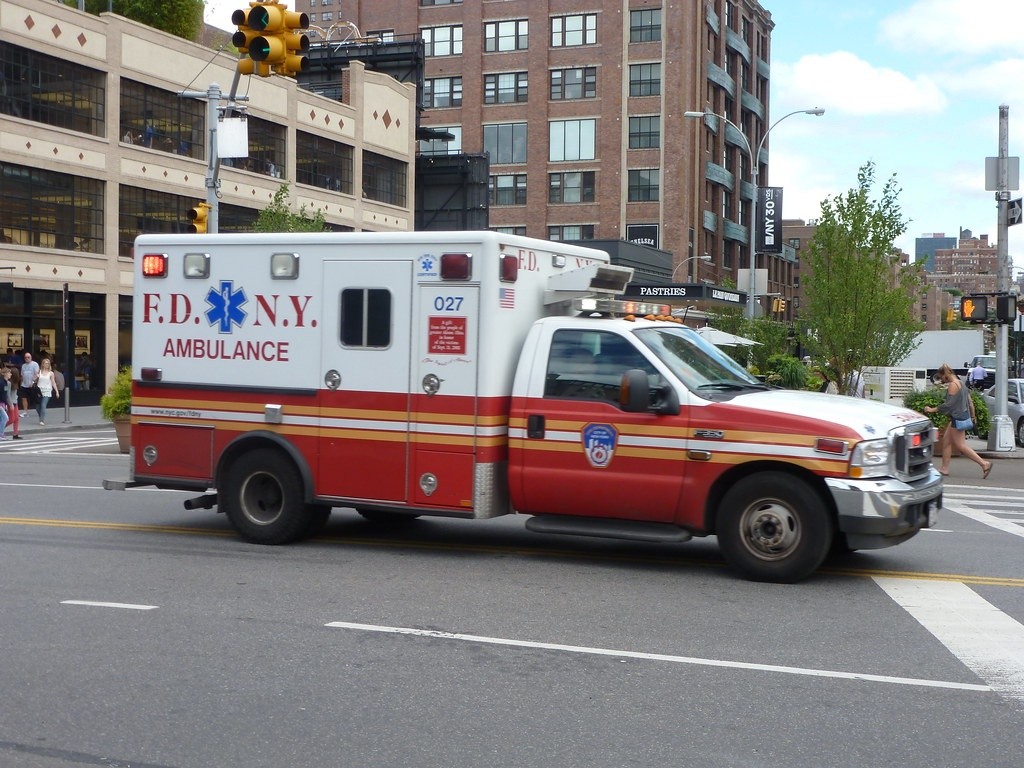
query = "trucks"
[846,331,984,386]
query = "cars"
[983,379,1024,449]
[964,355,996,389]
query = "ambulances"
[101,228,945,585]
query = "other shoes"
[0,436,8,441]
[19,412,28,417]
[40,421,45,426]
[13,435,22,440]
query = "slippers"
[938,470,949,476]
[983,463,993,479]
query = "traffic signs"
[1005,197,1022,228]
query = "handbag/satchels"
[955,417,973,431]
[29,387,42,403]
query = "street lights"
[669,254,712,283]
[685,101,826,335]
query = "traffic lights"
[961,296,988,321]
[773,299,787,313]
[229,7,256,75]
[947,310,956,324]
[1008,264,1024,275]
[187,204,205,234]
[285,8,311,75]
[249,3,286,64]
[996,296,1017,323]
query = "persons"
[123,121,153,149]
[160,136,188,155]
[923,363,994,479]
[262,158,275,178]
[0,348,65,441]
[820,354,866,399]
[560,348,607,398]
[969,362,988,389]
